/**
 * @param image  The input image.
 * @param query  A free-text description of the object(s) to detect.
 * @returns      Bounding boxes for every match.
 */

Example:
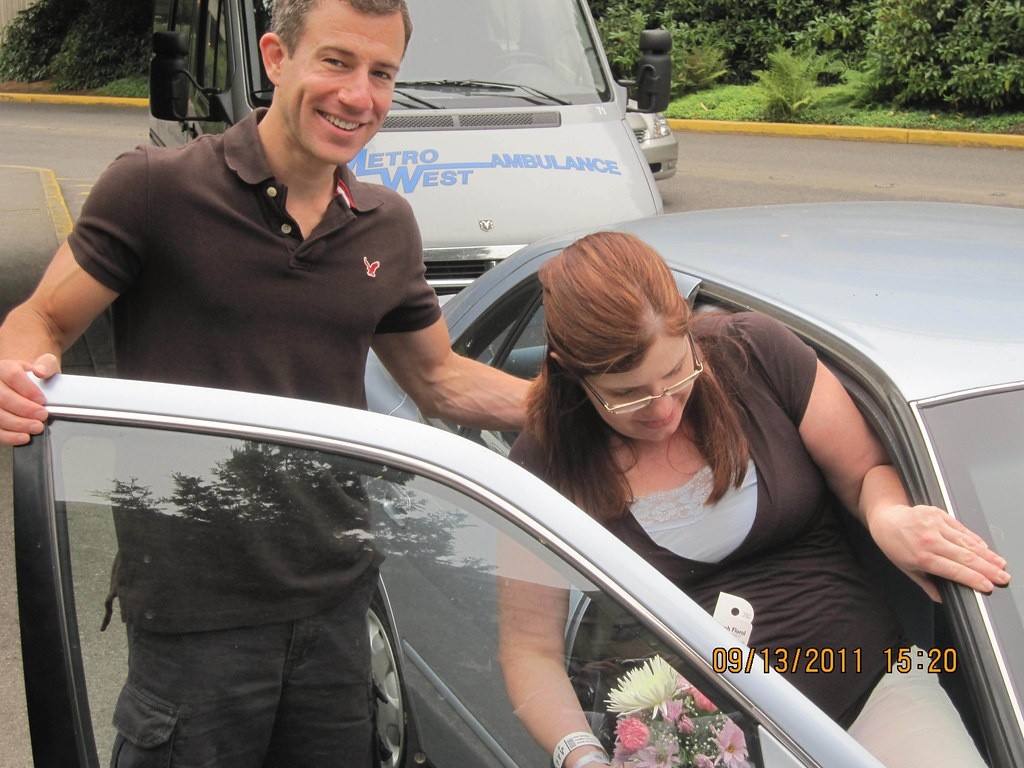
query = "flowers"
[604,651,752,768]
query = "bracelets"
[552,733,609,767]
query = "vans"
[149,0,665,383]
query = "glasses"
[571,326,705,414]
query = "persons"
[1,1,539,768]
[497,229,1012,768]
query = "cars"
[5,192,1024,768]
[623,111,680,183]
